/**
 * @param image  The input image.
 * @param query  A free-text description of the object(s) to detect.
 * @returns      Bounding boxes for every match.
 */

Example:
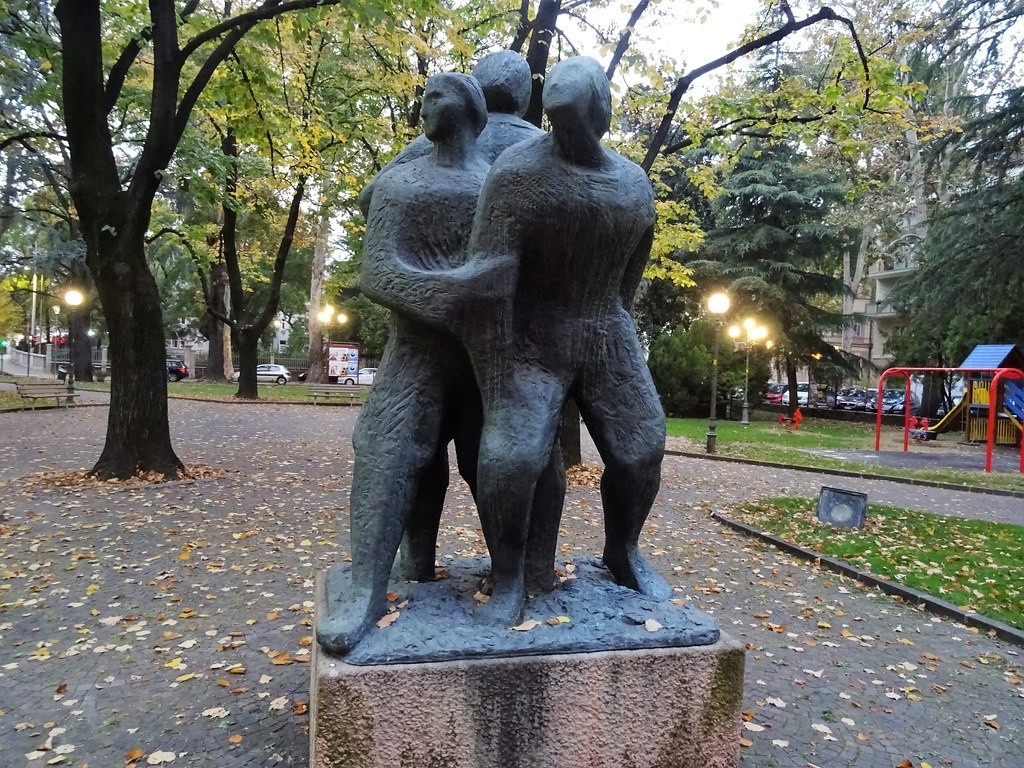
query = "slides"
[919,391,968,433]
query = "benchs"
[15,381,80,412]
[308,384,361,406]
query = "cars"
[763,384,788,404]
[165,359,190,382]
[865,388,920,414]
[828,388,872,411]
[298,367,337,382]
[337,367,378,387]
[782,382,809,405]
[936,402,956,419]
[232,364,292,386]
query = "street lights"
[727,317,769,425]
[51,304,61,378]
[318,303,347,379]
[706,293,730,454]
[64,290,84,403]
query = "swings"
[911,372,932,441]
[957,372,982,446]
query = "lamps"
[817,486,867,530]
